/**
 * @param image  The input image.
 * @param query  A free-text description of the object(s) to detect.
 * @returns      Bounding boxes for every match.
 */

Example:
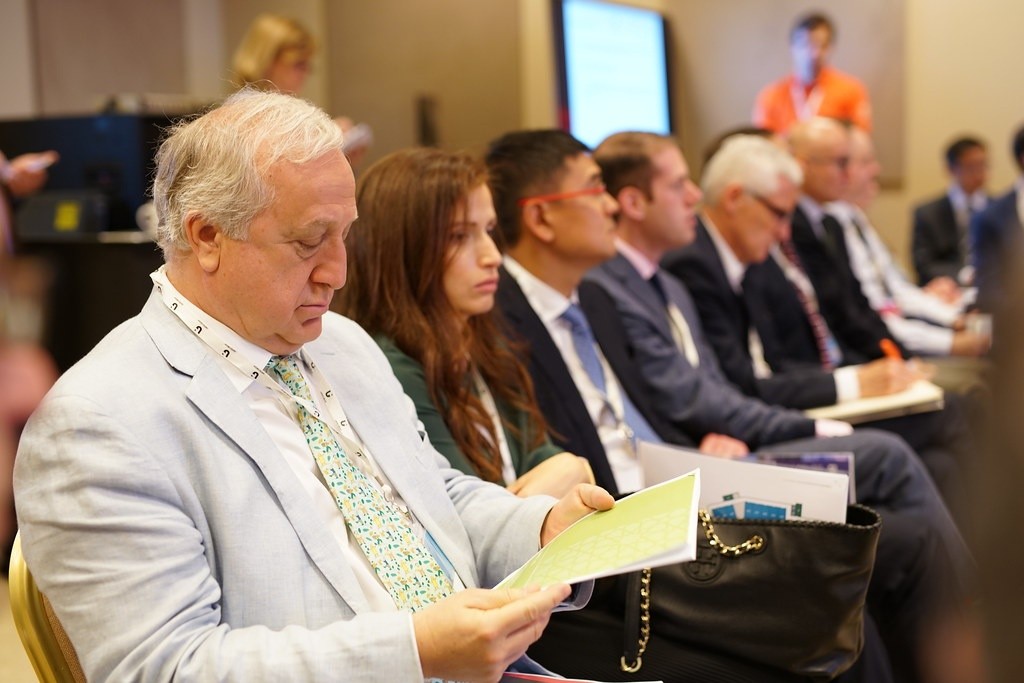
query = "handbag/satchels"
[601,488,882,683]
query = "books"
[804,380,945,424]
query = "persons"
[12,84,617,683]
[335,144,598,505]
[751,14,873,134]
[482,119,1024,683]
[233,12,354,133]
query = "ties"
[562,304,662,455]
[266,354,455,615]
[783,242,831,371]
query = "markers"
[878,337,901,358]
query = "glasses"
[522,182,611,206]
[813,153,851,169]
[752,194,797,223]
